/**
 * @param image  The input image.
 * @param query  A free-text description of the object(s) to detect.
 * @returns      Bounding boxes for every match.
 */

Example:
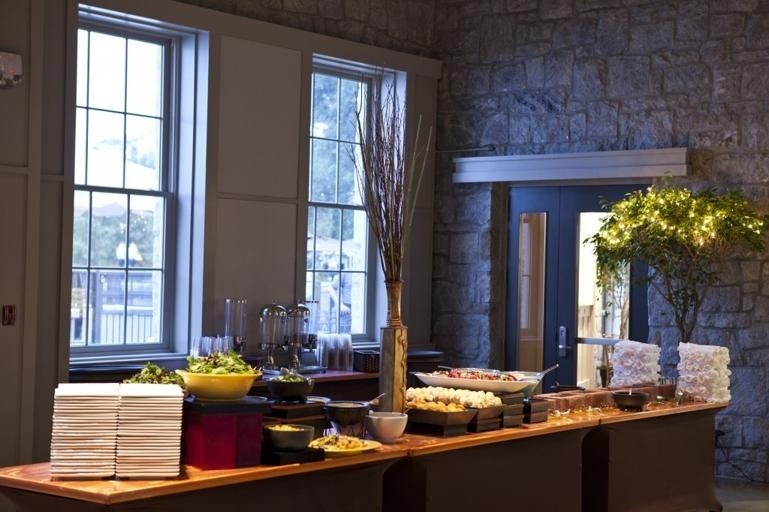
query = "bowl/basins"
[610,391,649,411]
[175,369,263,401]
[550,385,584,392]
[364,412,409,438]
[263,423,315,450]
[325,400,370,425]
[266,379,315,402]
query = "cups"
[190,335,234,358]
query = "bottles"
[317,334,353,371]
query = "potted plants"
[346,74,434,412]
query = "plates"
[50,383,184,477]
[409,372,537,393]
[307,440,382,455]
[366,435,409,443]
